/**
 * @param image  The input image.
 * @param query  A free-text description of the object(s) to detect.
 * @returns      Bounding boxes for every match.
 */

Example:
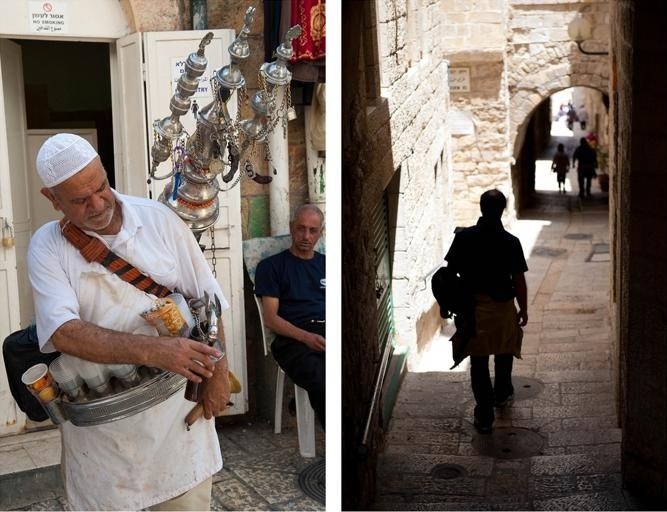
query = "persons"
[254,203,324,435]
[25,131,231,511]
[440,188,531,436]
[585,132,598,145]
[571,136,597,198]
[549,143,570,194]
[567,105,576,131]
[577,103,589,130]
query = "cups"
[21,292,221,426]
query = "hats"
[36,131,99,190]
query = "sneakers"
[494,390,515,409]
[473,416,492,433]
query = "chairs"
[253,286,315,458]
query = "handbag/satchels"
[431,228,471,317]
